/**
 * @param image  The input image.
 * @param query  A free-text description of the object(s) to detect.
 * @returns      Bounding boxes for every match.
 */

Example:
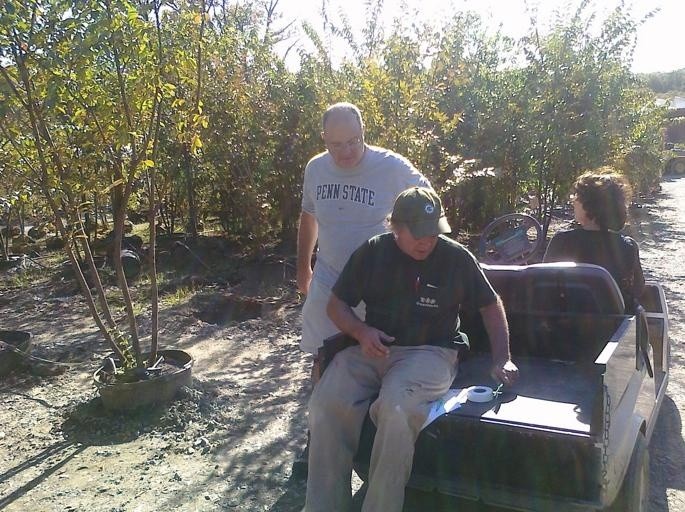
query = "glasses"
[324,132,364,150]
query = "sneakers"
[293,430,308,469]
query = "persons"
[291,101,450,481]
[542,165,647,315]
[300,188,520,511]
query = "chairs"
[504,260,651,360]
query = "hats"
[390,186,451,238]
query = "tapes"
[466,386,493,402]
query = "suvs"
[315,212,672,512]
[664,150,685,176]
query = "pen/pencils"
[415,276,421,295]
